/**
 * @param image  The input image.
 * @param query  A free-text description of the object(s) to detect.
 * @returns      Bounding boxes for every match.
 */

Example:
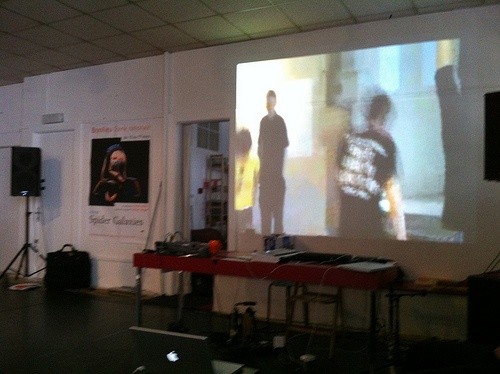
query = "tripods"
[0,196,47,280]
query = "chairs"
[284,282,343,358]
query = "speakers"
[483,91,500,182]
[11,147,42,196]
[45,252,90,291]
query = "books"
[252,248,302,263]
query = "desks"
[133,252,401,360]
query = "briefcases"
[45,245,91,289]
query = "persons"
[257,91,289,234]
[92,144,145,206]
[235,129,260,233]
[336,96,406,240]
